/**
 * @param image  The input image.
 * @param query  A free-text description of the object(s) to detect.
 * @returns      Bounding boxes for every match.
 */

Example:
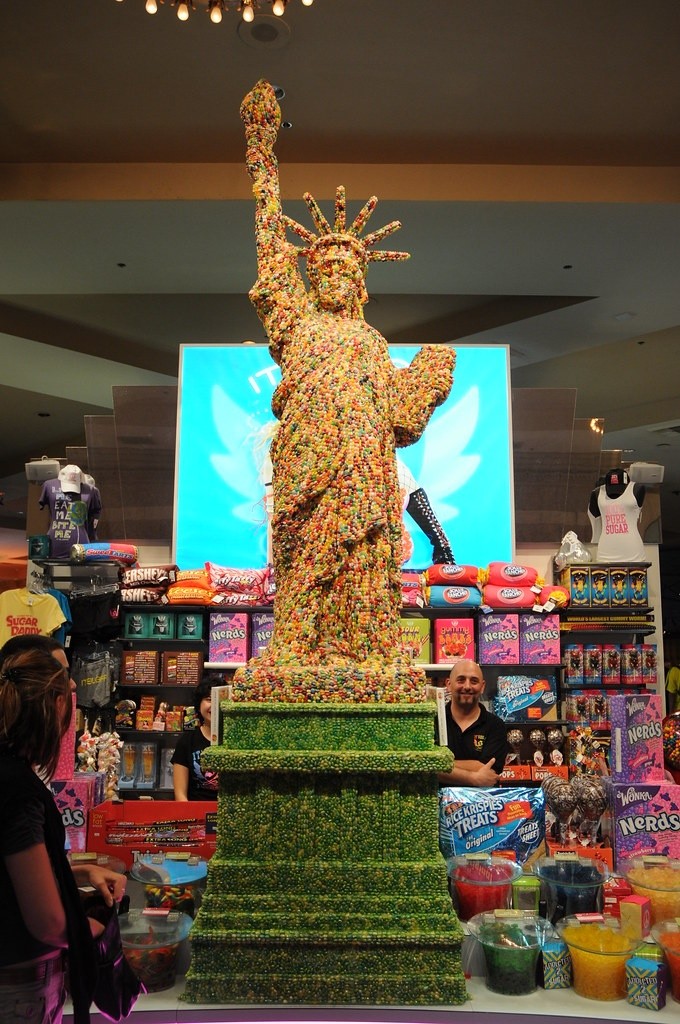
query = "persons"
[39,465,102,559]
[170,678,230,803]
[431,659,508,787]
[0,633,126,1024]
[587,469,646,562]
[241,80,455,668]
[665,656,680,713]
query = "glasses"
[66,668,71,680]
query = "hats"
[57,465,84,494]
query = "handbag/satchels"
[83,883,144,1023]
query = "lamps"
[115,0,314,23]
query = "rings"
[122,889,126,893]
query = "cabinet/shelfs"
[110,598,569,798]
[552,555,658,738]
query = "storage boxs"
[511,876,540,910]
[539,941,572,990]
[625,956,667,1012]
[602,879,631,918]
[49,566,680,872]
[619,894,654,939]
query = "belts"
[0,955,64,986]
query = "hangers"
[19,572,119,671]
